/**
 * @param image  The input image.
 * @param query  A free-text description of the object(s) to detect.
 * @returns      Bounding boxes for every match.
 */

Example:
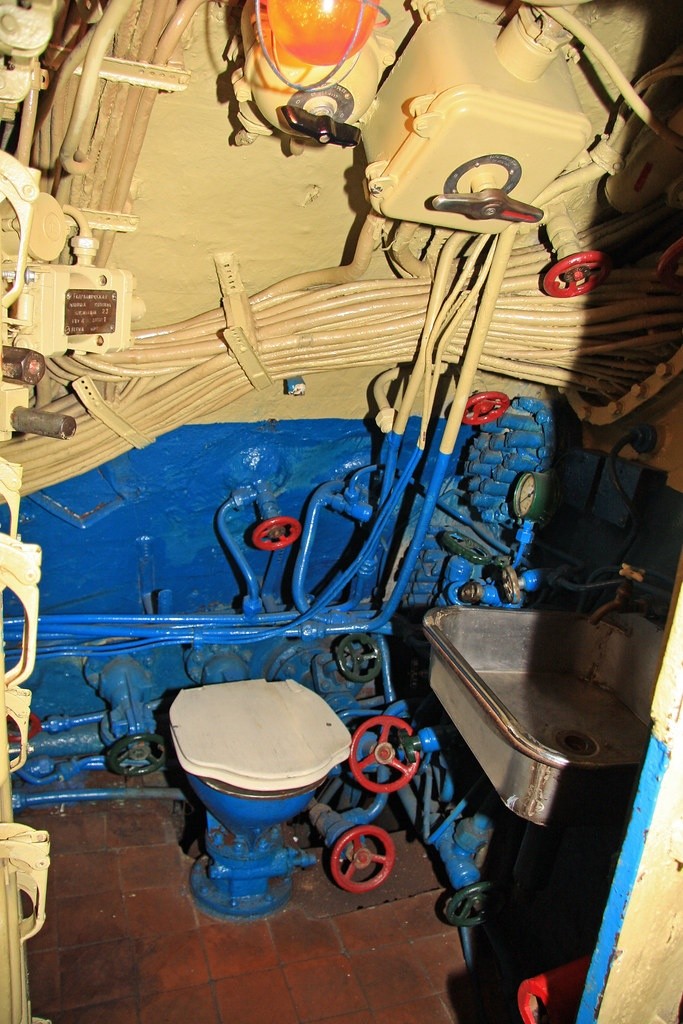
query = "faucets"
[588,563,667,632]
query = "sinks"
[421,604,669,830]
